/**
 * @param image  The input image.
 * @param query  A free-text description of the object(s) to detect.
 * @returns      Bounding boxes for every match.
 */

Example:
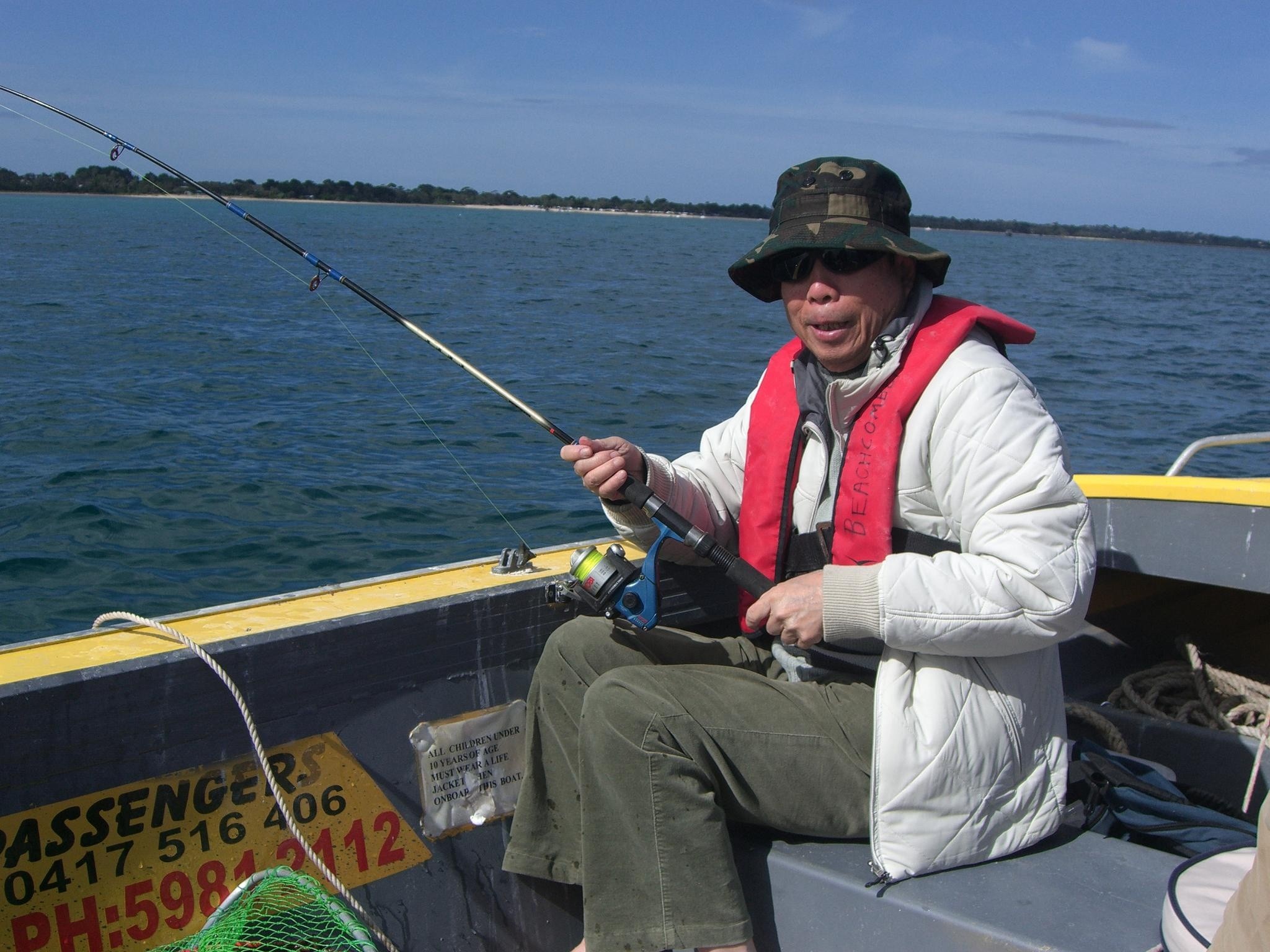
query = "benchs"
[728,818,1188,952]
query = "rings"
[782,644,796,647]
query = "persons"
[500,157,1099,952]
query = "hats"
[730,155,951,303]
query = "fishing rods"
[1,85,777,632]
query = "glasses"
[769,247,884,284]
